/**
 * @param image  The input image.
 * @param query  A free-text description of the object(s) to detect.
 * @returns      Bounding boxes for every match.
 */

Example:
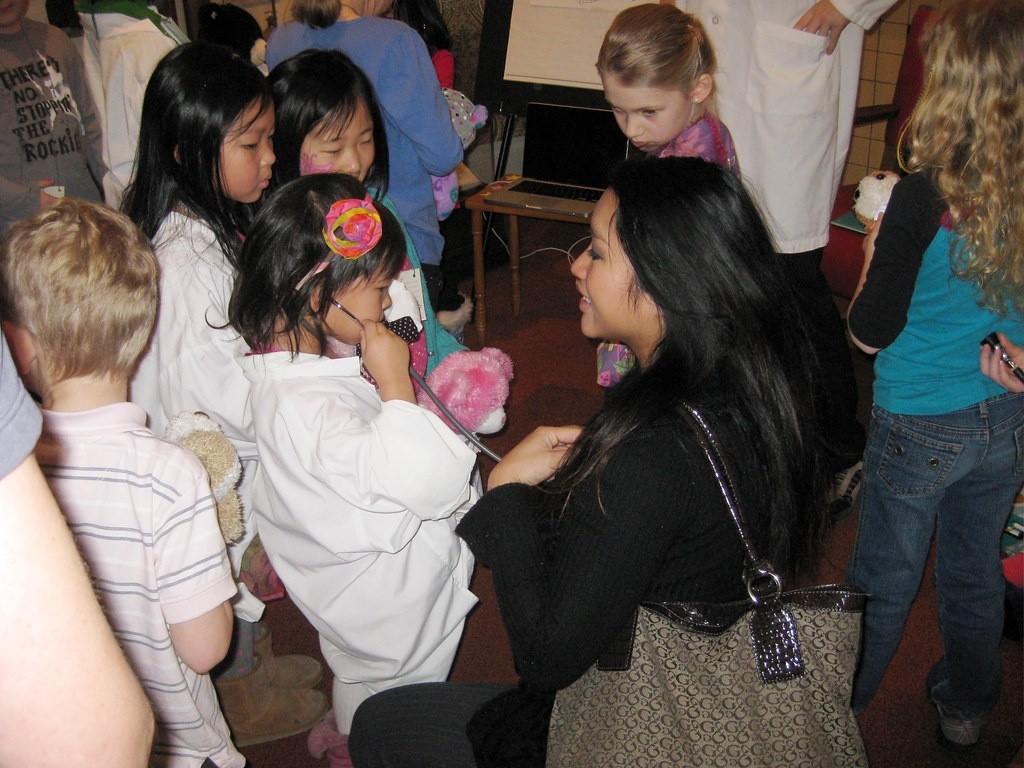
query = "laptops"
[483,103,630,218]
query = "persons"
[347,156,832,768]
[229,172,482,768]
[662,0,897,525]
[0,196,246,768]
[0,1,466,344]
[267,48,471,381]
[981,332,1024,393]
[117,42,330,748]
[843,0,1023,747]
[596,4,740,401]
[0,326,155,767]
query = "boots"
[213,627,329,747]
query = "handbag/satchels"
[545,397,869,768]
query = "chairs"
[821,4,989,300]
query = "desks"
[466,173,597,347]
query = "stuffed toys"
[159,412,245,545]
[417,347,513,453]
[852,169,902,233]
[431,86,488,220]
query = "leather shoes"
[435,290,474,335]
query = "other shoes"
[938,707,984,745]
